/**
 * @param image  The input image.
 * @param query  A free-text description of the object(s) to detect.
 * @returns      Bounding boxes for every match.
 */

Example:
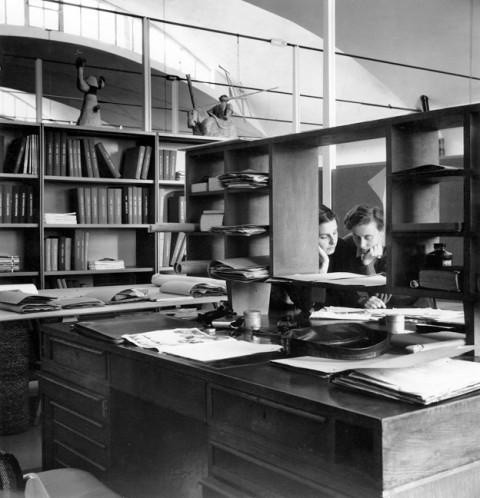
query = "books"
[0,130,223,289]
[74,313,205,346]
[418,264,464,293]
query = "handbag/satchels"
[280,322,391,360]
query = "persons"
[319,204,356,308]
[342,203,392,309]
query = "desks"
[1,283,229,436]
[38,311,480,497]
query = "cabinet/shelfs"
[271,119,388,311]
[1,123,41,293]
[390,108,477,358]
[158,132,222,272]
[185,136,270,310]
[42,124,158,289]
